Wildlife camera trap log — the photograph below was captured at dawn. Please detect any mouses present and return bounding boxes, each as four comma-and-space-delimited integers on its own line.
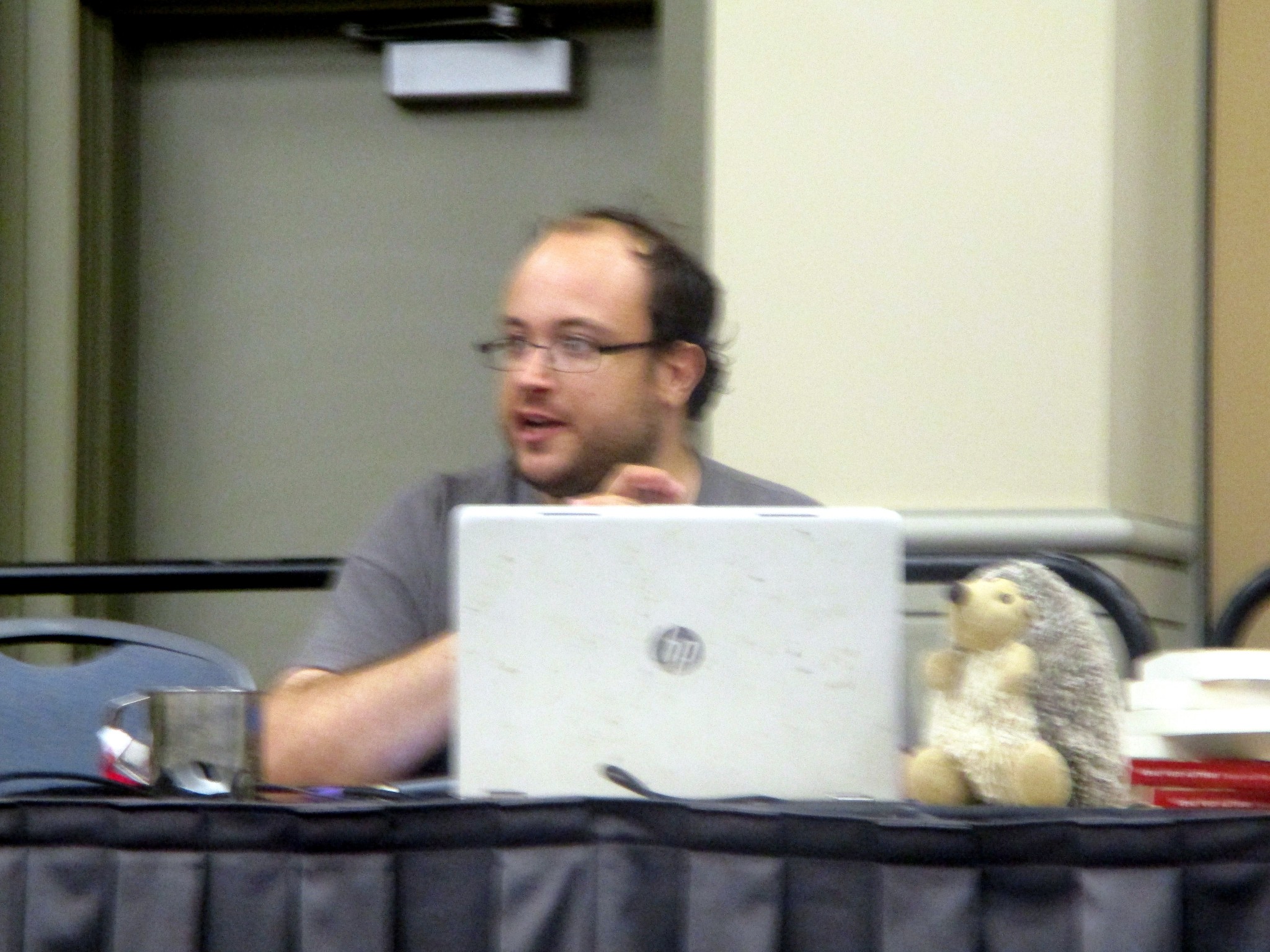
159, 761, 232, 797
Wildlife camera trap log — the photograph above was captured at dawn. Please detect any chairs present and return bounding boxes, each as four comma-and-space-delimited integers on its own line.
0, 615, 258, 794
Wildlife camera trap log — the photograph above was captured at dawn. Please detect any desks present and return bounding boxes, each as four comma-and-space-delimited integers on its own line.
0, 775, 1270, 952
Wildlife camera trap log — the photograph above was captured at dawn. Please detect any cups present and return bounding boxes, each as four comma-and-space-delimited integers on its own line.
105, 687, 256, 794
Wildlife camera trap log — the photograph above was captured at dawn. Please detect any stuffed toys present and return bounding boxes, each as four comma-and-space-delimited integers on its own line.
900, 561, 1134, 814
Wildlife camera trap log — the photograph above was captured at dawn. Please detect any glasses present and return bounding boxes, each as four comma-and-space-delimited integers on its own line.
468, 331, 656, 374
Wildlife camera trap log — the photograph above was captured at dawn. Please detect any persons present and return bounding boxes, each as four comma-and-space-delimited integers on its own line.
255, 207, 917, 804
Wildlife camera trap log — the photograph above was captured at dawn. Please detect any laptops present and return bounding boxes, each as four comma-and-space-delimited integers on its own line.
424, 500, 908, 806
